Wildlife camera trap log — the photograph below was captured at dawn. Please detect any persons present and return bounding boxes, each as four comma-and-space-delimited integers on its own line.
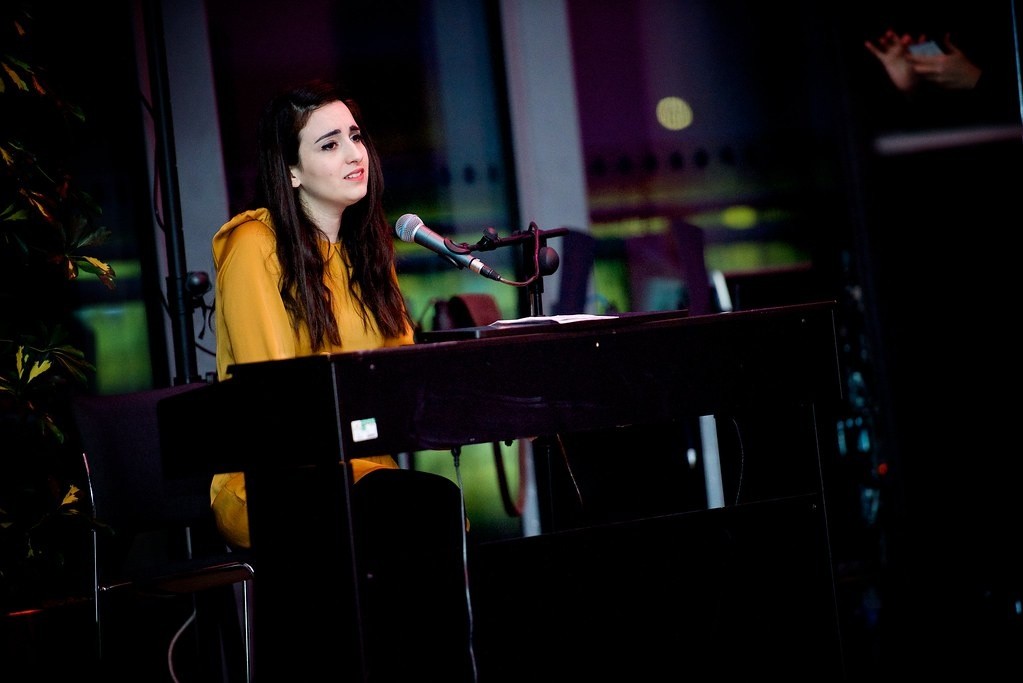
210, 79, 469, 549
857, 1, 1023, 614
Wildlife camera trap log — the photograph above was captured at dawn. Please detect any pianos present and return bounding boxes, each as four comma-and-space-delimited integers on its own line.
145, 294, 867, 464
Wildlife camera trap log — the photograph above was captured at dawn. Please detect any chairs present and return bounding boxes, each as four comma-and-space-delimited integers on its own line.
75, 381, 254, 683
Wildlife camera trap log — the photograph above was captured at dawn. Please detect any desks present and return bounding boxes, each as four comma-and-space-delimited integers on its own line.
166, 296, 843, 683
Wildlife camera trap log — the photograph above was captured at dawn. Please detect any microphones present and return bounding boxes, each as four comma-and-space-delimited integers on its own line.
395, 214, 501, 281
